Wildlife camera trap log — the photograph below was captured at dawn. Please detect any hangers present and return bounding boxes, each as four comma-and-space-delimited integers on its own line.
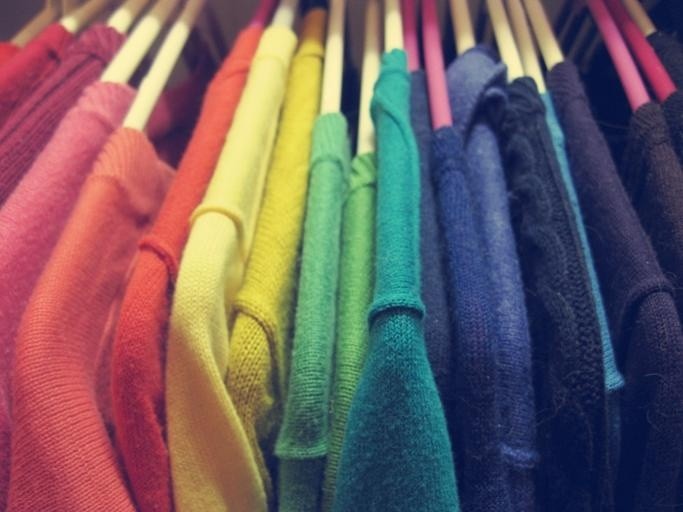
0, 0, 683, 174
